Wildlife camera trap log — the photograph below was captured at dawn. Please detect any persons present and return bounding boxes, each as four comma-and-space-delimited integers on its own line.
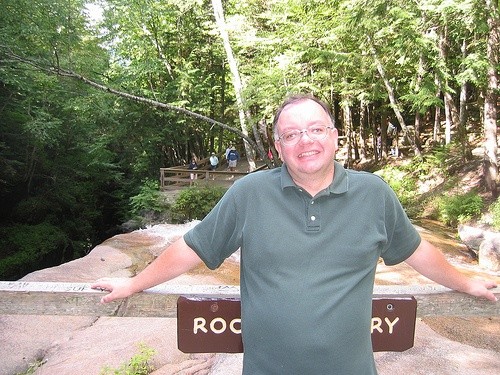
90, 93, 499, 375
225, 144, 233, 166
188, 159, 199, 182
227, 147, 238, 175
377, 121, 396, 156
208, 151, 218, 176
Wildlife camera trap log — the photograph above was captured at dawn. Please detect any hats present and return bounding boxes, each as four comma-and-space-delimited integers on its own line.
212, 152, 215, 155
230, 147, 236, 150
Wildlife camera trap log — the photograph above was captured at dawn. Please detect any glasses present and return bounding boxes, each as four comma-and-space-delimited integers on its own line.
278, 124, 334, 145
